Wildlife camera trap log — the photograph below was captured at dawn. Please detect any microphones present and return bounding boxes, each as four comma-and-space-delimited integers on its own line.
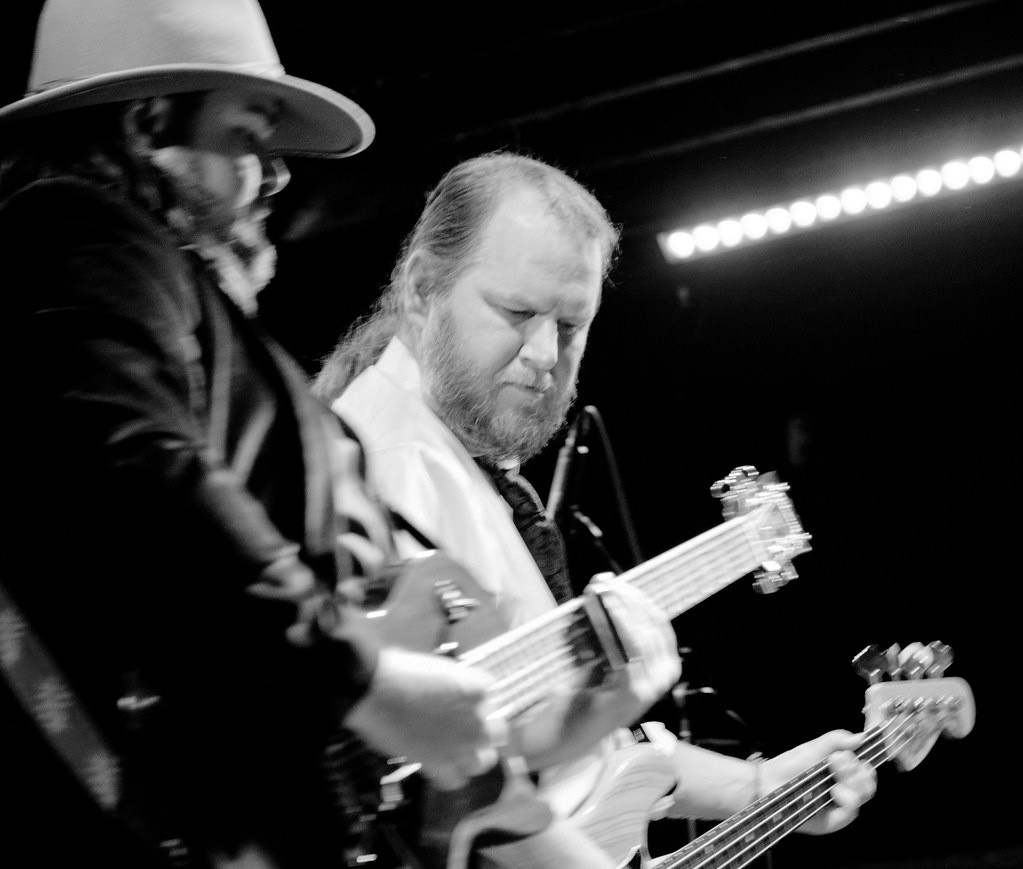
546, 411, 593, 538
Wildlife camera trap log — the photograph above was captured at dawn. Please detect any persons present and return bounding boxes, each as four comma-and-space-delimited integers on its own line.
1, 0, 683, 868
309, 151, 879, 869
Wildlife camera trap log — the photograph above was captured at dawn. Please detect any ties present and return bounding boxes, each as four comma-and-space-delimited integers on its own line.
472, 456, 575, 606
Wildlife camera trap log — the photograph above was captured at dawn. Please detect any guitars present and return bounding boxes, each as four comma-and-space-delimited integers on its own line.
117, 463, 814, 869
567, 637, 976, 869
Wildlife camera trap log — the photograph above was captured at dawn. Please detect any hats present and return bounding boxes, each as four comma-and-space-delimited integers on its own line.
0, 1, 376, 160
